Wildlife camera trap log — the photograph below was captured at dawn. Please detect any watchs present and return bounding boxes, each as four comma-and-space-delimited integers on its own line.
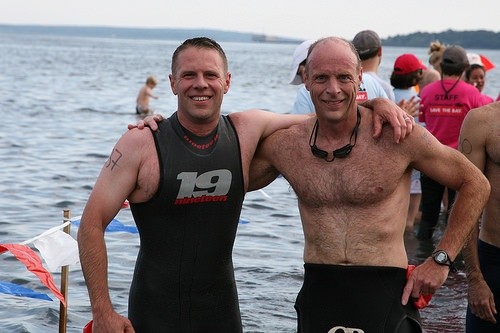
430, 249, 454, 268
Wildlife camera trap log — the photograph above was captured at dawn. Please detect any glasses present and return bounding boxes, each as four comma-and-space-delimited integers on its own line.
310, 143, 355, 159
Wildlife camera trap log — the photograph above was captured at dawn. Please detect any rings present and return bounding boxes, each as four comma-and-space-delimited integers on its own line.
404, 116, 410, 120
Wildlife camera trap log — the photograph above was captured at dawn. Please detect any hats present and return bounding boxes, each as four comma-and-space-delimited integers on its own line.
440, 44, 471, 70
392, 53, 427, 76
466, 53, 485, 67
352, 29, 381, 55
288, 39, 318, 85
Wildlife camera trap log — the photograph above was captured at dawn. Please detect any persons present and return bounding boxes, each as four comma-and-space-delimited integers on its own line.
76, 38, 415, 333
136, 76, 159, 115
127, 37, 493, 333
287, 30, 500, 333
